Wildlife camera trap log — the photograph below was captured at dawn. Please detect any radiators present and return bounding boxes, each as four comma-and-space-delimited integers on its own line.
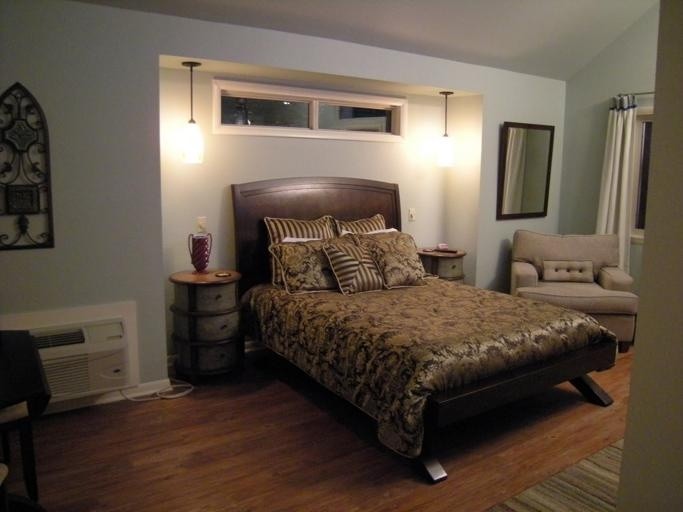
0, 316, 138, 405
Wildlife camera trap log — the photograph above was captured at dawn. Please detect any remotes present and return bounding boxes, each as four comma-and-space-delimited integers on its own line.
434, 248, 457, 253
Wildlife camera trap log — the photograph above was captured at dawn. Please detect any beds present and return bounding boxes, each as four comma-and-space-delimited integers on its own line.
232, 176, 619, 482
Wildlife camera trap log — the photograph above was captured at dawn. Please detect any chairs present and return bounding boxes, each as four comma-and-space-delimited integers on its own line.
510, 228, 639, 353
1, 329, 52, 512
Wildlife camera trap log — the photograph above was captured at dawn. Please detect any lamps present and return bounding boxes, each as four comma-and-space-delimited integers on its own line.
428, 91, 455, 167
165, 60, 203, 165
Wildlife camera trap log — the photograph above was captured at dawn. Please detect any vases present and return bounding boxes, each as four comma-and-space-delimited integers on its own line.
187, 232, 212, 275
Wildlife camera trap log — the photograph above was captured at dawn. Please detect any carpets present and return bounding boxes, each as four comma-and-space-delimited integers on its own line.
483, 438, 625, 512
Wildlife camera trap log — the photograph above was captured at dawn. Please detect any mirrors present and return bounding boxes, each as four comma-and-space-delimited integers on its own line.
496, 122, 554, 220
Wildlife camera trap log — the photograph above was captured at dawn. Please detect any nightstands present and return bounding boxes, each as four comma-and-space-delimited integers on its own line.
169, 271, 240, 391
417, 245, 466, 282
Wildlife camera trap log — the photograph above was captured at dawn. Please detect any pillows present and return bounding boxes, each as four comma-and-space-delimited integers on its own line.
543, 259, 595, 283
347, 230, 418, 247
368, 236, 427, 289
268, 237, 354, 295
322, 243, 387, 296
333, 213, 386, 237
265, 215, 341, 289
